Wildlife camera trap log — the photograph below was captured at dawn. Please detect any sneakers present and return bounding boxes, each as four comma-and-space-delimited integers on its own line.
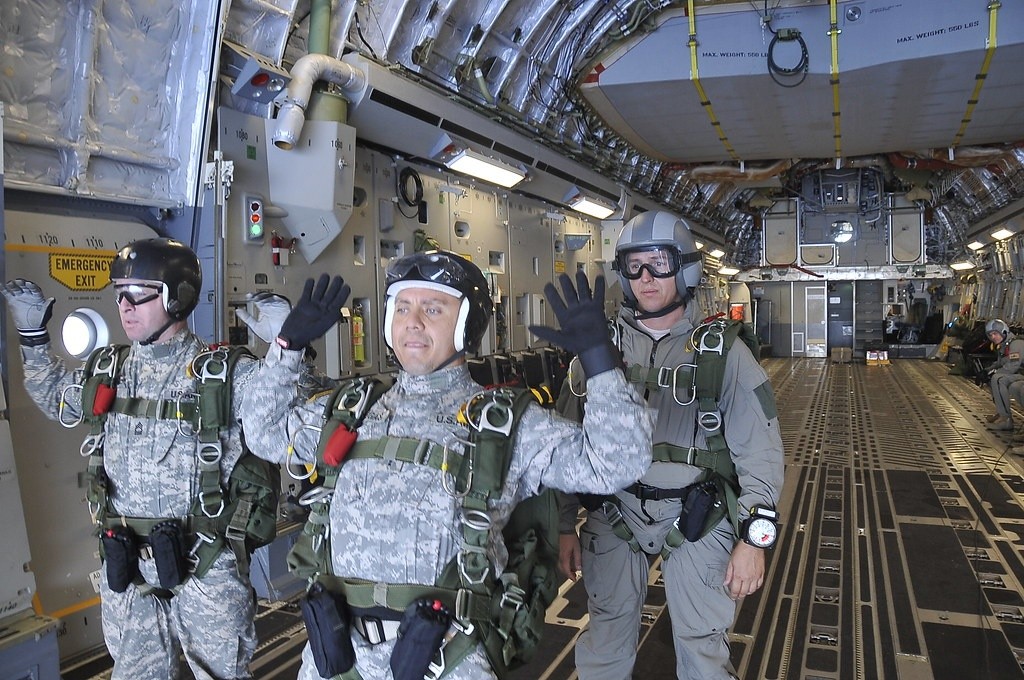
985, 414, 1014, 430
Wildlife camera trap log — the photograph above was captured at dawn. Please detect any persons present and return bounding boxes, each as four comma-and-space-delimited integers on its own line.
977, 319, 1023, 429
240, 252, 654, 679
0, 238, 293, 679
557, 211, 784, 680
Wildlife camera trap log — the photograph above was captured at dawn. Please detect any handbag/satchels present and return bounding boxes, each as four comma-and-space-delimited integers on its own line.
150, 520, 188, 590
299, 585, 356, 680
678, 477, 729, 543
98, 524, 139, 593
389, 598, 452, 680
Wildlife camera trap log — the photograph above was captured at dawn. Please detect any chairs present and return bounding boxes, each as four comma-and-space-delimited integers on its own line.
970, 353, 997, 370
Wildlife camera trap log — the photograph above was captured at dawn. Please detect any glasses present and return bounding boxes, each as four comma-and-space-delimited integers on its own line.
114, 283, 163, 306
385, 254, 495, 314
618, 244, 680, 280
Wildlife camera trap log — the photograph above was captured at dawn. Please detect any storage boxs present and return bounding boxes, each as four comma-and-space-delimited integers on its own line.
830, 347, 852, 362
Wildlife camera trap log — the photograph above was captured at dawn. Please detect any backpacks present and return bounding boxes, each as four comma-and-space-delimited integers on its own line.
78, 341, 281, 551
285, 374, 563, 680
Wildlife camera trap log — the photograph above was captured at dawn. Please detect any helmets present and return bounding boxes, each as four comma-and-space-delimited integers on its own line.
613, 211, 703, 303
985, 319, 1010, 341
109, 238, 203, 323
382, 249, 494, 355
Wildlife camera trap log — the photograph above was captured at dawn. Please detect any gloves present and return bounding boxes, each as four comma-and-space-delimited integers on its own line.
278, 273, 351, 352
528, 271, 624, 380
0, 278, 57, 346
235, 291, 318, 360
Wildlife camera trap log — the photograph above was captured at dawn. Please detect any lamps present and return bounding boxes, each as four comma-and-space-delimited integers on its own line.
569, 196, 615, 219
717, 266, 740, 276
445, 148, 526, 188
949, 260, 977, 270
